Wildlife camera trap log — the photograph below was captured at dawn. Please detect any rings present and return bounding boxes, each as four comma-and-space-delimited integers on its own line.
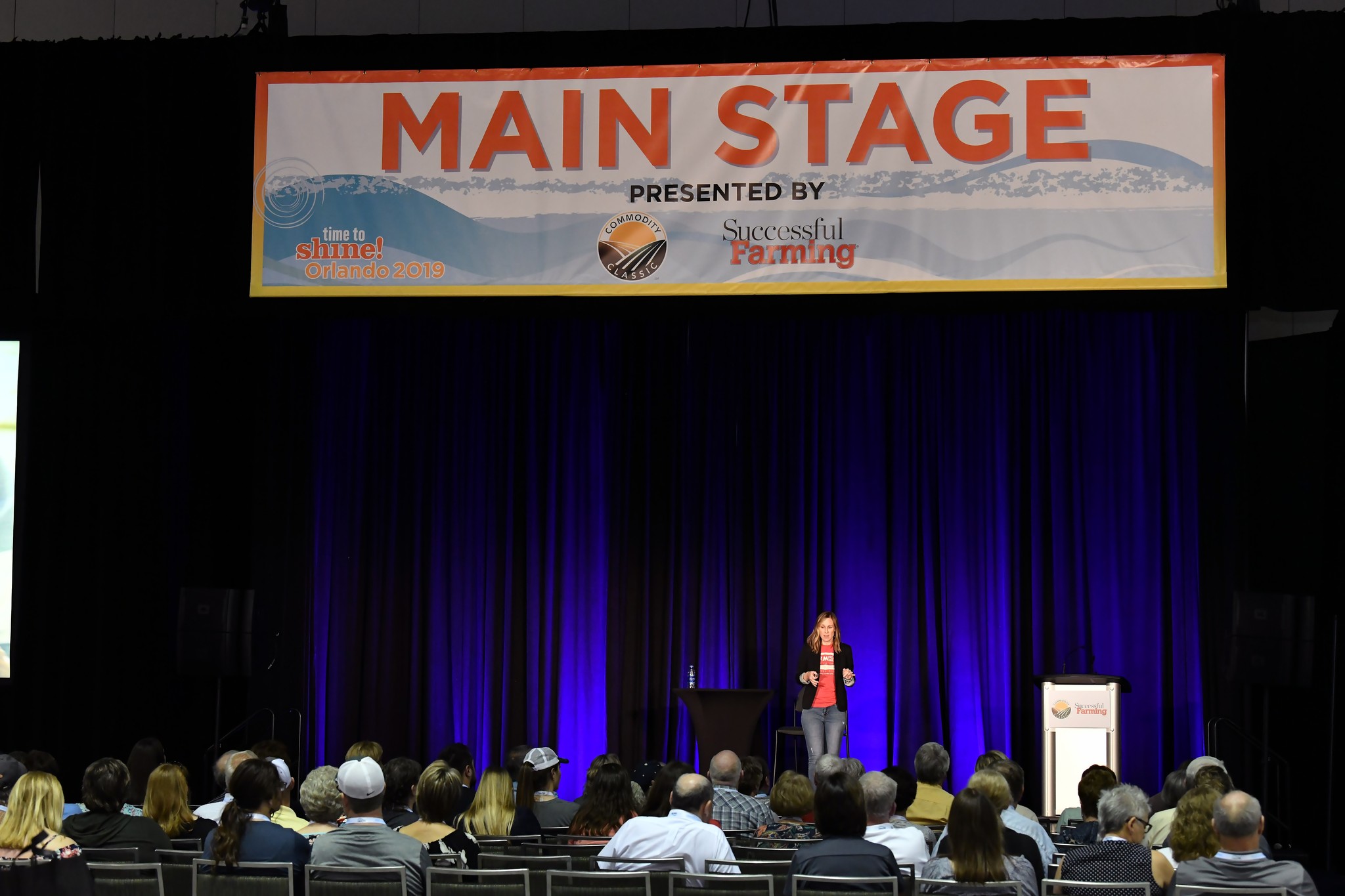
851, 672, 853, 674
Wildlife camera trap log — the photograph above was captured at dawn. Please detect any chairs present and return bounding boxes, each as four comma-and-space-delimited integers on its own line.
0, 808, 1290, 896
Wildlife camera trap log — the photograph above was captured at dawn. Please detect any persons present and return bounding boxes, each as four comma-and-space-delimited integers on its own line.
0, 741, 308, 896
568, 741, 1061, 896
1051, 754, 1319, 896
795, 612, 856, 790
296, 739, 580, 896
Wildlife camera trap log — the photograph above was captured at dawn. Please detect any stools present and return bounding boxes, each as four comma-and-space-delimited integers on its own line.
771, 701, 851, 787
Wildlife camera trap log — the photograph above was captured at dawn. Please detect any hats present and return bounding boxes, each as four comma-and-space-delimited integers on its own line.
264, 756, 291, 790
335, 756, 385, 799
523, 747, 570, 771
1186, 756, 1228, 781
0, 754, 26, 790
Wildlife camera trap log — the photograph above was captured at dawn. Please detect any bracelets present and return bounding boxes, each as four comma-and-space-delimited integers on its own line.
844, 677, 853, 683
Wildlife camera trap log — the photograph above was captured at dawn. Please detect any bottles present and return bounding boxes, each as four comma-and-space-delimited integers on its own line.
688, 665, 695, 689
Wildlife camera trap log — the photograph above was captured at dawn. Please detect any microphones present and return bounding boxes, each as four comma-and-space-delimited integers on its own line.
1063, 646, 1085, 674
268, 659, 275, 669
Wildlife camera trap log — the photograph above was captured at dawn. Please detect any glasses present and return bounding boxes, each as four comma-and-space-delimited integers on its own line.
159, 761, 183, 769
1126, 817, 1153, 833
276, 781, 287, 791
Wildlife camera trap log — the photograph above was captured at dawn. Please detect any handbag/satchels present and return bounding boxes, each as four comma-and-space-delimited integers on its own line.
0, 830, 94, 896
